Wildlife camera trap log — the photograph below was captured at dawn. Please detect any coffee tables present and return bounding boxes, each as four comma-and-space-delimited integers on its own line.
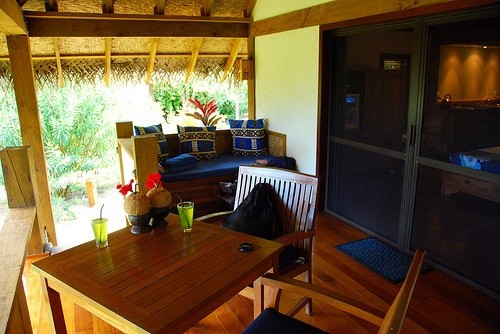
443, 146, 500, 202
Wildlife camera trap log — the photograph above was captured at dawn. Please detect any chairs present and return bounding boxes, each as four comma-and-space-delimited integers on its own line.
195, 164, 320, 317
242, 249, 427, 334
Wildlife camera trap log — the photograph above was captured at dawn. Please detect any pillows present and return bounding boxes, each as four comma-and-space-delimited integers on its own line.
166, 154, 197, 172
176, 125, 218, 161
134, 123, 169, 160
228, 118, 268, 157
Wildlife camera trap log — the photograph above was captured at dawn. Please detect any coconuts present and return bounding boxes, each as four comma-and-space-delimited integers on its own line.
146, 186, 171, 208
124, 191, 151, 217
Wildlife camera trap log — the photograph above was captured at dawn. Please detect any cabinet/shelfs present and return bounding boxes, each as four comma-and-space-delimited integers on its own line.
348, 70, 405, 167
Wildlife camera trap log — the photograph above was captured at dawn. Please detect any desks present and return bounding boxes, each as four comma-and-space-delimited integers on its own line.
32, 213, 283, 334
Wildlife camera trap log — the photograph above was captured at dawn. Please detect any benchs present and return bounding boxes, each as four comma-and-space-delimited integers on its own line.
440, 107, 500, 150
116, 122, 298, 209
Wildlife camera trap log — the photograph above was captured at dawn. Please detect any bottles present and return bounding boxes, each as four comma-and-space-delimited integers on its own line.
436, 97, 442, 108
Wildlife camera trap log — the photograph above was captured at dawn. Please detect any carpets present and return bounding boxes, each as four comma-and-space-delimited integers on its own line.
333, 236, 438, 284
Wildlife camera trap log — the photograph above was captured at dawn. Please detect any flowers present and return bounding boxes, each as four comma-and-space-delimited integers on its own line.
145, 173, 163, 189
117, 179, 135, 194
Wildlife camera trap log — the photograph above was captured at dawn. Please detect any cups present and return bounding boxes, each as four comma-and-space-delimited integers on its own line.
177, 202, 194, 232
90, 217, 109, 248
